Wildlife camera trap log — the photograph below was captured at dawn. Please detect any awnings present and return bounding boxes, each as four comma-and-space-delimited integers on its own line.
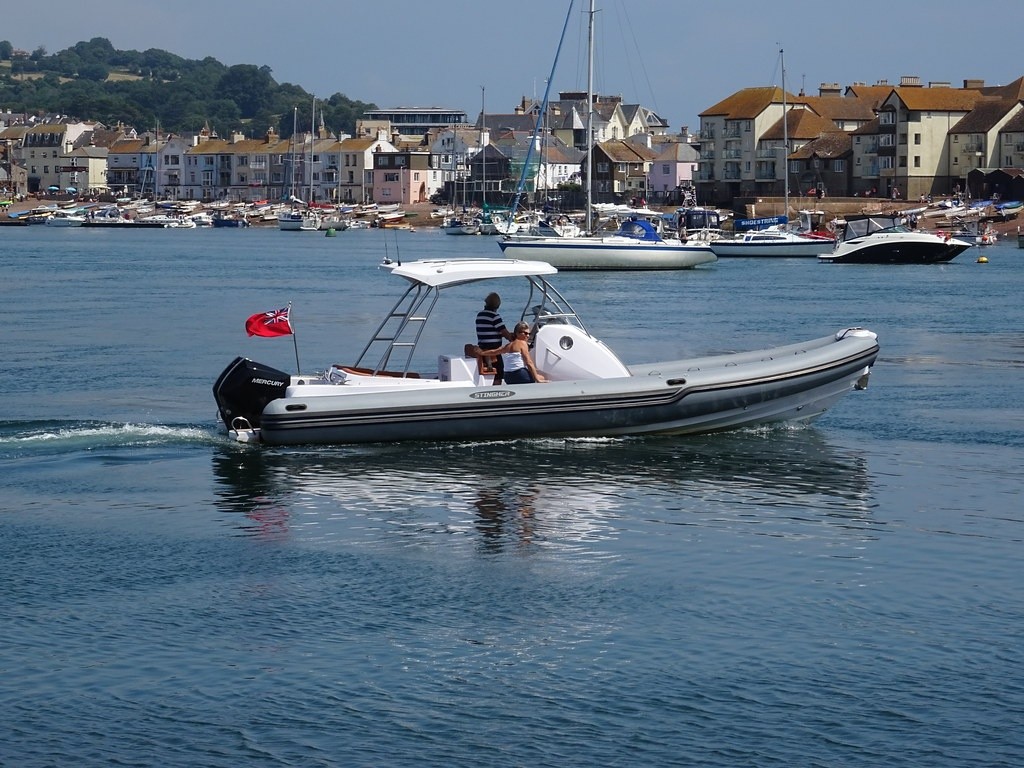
164, 170, 178, 177
106, 167, 138, 171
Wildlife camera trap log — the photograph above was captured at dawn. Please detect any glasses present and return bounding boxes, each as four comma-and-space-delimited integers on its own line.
519, 331, 531, 336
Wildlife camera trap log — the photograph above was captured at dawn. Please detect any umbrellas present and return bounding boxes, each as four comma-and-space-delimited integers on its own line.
99, 185, 111, 194
49, 185, 59, 194
123, 185, 129, 197
66, 187, 77, 195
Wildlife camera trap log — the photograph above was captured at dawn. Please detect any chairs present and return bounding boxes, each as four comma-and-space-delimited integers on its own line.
466, 344, 498, 375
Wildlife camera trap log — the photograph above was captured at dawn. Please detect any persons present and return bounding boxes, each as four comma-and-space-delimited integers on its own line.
892, 190, 897, 201
863, 186, 866, 198
473, 321, 550, 384
954, 183, 962, 206
2, 186, 15, 214
475, 292, 514, 385
91, 209, 94, 219
93, 187, 99, 196
893, 209, 917, 229
927, 193, 931, 203
872, 187, 877, 198
115, 189, 123, 198
920, 192, 925, 204
815, 187, 822, 202
640, 197, 646, 208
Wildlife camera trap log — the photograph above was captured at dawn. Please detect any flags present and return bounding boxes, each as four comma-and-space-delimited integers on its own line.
246, 312, 293, 338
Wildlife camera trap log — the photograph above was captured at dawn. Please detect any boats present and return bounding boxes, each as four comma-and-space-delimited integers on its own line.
430, 0, 1024, 268
0, 94, 417, 230
212, 233, 881, 447
